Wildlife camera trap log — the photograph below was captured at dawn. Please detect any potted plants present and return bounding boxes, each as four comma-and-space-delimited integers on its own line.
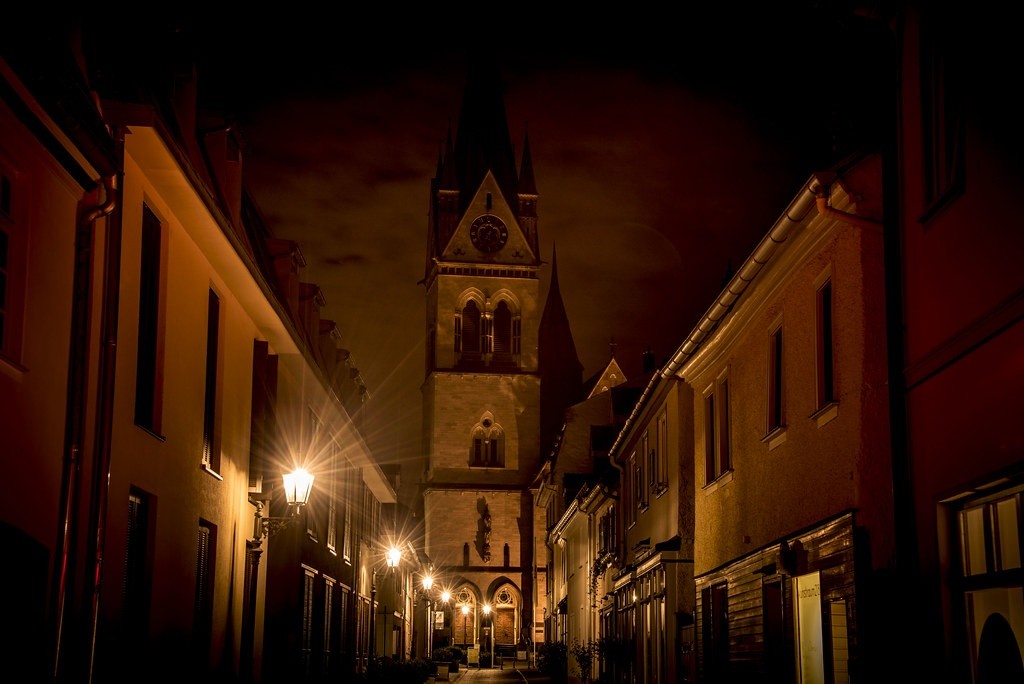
432, 648, 453, 680
444, 646, 465, 673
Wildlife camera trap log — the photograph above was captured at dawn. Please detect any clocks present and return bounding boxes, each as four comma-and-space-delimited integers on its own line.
471, 216, 508, 253
